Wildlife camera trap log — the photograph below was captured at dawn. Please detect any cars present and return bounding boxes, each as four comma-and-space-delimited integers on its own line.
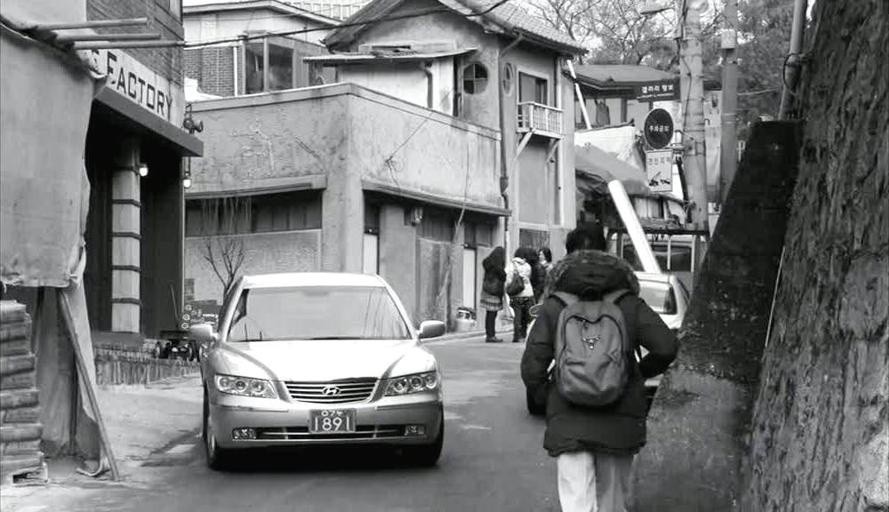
187, 268, 446, 468
521, 267, 691, 418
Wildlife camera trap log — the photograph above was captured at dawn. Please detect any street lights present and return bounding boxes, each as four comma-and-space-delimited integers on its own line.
634, 3, 711, 256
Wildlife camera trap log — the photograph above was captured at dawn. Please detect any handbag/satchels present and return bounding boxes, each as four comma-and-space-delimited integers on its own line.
507, 273, 524, 296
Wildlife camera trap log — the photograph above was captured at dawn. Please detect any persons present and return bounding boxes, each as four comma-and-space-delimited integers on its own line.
479, 247, 553, 341
521, 227, 678, 512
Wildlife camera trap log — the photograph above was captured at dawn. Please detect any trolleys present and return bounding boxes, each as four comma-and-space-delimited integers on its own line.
153, 282, 197, 362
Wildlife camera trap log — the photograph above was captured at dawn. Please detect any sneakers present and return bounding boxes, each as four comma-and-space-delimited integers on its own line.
486, 336, 501, 342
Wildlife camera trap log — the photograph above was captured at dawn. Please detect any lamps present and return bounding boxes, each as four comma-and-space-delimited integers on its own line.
183, 103, 205, 135
128, 137, 149, 179
179, 150, 195, 193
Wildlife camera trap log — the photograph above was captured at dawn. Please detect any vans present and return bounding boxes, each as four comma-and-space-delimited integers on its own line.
614, 229, 692, 297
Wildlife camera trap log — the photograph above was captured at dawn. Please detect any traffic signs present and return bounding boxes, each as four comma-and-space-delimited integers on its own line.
633, 78, 680, 105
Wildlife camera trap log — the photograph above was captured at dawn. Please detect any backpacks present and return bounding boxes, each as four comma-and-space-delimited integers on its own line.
549, 289, 631, 406
